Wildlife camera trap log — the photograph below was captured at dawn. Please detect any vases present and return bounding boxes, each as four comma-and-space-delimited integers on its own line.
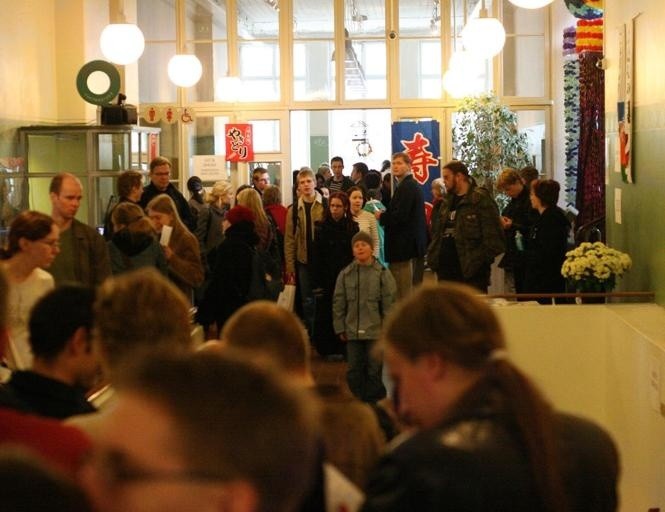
561, 241, 633, 286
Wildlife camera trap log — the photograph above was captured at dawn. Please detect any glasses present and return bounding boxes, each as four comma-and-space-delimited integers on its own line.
167, 47, 202, 88
443, 0, 506, 99
98, 14, 145, 66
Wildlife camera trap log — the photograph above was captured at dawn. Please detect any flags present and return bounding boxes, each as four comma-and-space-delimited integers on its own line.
578, 278, 609, 303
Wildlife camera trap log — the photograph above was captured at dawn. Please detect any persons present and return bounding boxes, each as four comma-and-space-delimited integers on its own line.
1, 281, 101, 421
80, 349, 314, 510
103, 153, 572, 396
2, 443, 94, 512
2, 209, 61, 369
223, 300, 386, 492
39, 170, 114, 289
358, 281, 621, 510
62, 272, 194, 435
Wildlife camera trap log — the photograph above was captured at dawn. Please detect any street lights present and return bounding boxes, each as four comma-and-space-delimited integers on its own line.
278, 283, 296, 313
425, 233, 459, 275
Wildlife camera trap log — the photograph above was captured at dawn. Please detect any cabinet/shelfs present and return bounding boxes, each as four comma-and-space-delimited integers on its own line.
515, 230, 523, 252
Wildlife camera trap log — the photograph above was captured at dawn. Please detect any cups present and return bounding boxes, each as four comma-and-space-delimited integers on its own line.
151, 171, 170, 176
